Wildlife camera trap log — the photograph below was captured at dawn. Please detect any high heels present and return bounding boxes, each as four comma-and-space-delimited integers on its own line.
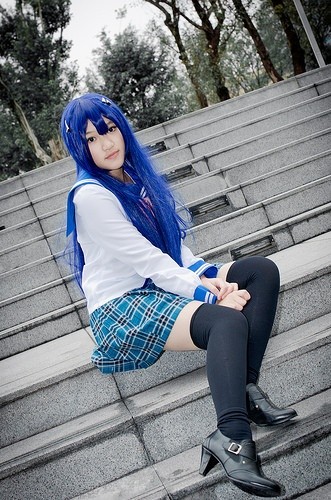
245, 382, 297, 428
198, 428, 281, 498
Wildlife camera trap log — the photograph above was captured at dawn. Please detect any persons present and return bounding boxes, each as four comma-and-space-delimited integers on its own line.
59, 91, 301, 497
